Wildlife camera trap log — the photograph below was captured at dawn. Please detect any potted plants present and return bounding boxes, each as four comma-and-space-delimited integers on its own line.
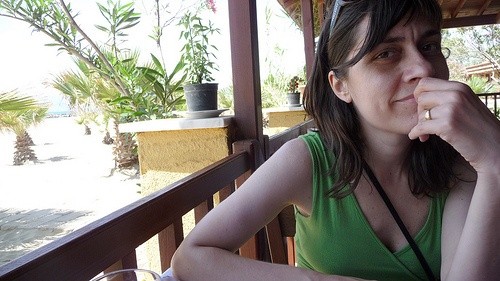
284, 76, 304, 104
177, 11, 222, 111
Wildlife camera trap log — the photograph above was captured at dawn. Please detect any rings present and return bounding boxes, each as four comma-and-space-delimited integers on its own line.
425, 110, 432, 120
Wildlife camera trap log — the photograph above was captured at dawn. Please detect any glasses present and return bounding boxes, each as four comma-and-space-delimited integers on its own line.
329, 0, 356, 39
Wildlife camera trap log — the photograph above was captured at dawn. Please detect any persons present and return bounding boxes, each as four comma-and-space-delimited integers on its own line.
171, 0, 500, 281
